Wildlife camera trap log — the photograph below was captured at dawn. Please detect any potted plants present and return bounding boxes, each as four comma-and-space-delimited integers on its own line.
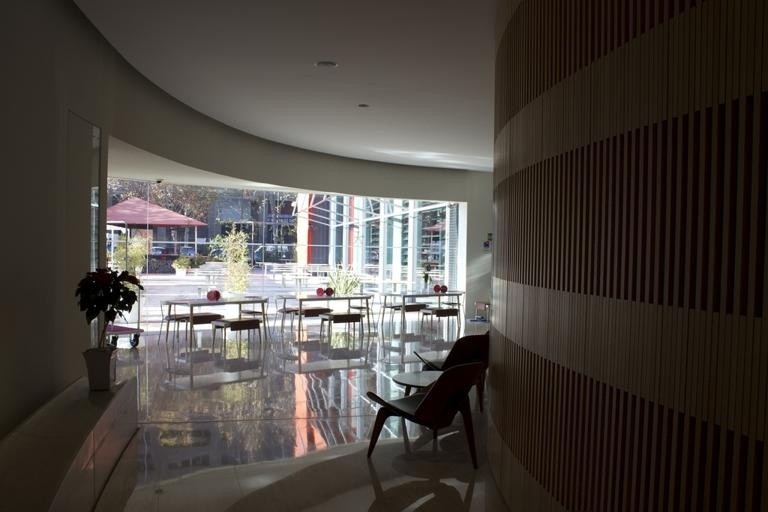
171, 255, 191, 276
74, 267, 144, 391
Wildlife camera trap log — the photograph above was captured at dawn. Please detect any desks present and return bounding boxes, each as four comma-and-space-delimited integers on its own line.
474, 299, 490, 322
196, 259, 440, 293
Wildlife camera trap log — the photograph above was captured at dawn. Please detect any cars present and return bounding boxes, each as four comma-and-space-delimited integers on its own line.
209, 248, 224, 256
181, 247, 194, 255
151, 246, 164, 255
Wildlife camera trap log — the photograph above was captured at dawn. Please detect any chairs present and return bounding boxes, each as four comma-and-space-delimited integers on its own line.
380, 331, 459, 358
239, 296, 269, 330
417, 329, 490, 410
366, 362, 482, 470
392, 335, 484, 448
377, 291, 463, 331
273, 334, 376, 374
157, 299, 188, 345
273, 293, 374, 333
161, 344, 269, 391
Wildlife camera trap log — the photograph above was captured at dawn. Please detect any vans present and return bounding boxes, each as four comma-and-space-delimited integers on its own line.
254, 243, 293, 263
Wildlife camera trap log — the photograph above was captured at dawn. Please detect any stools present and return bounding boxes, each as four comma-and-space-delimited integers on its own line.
211, 319, 262, 347
165, 312, 224, 340
